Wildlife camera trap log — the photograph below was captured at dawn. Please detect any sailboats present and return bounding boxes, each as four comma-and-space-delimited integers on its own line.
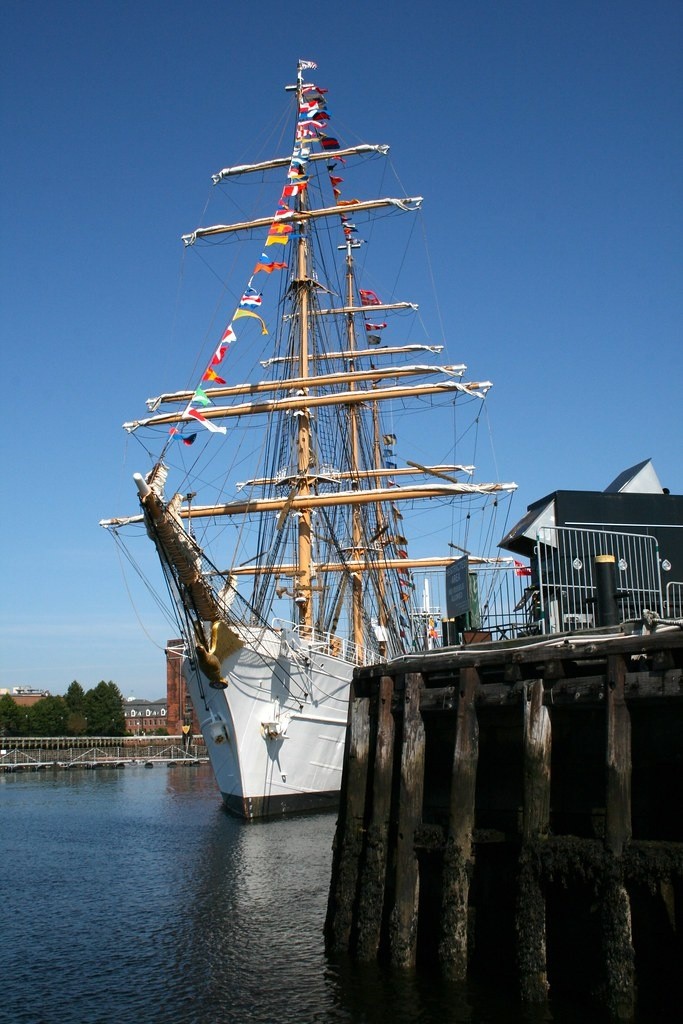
96, 58, 541, 821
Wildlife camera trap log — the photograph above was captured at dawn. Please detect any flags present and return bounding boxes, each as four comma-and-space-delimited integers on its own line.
300, 60, 318, 71
372, 368, 382, 384
167, 253, 289, 447
366, 322, 387, 331
430, 629, 438, 639
385, 461, 397, 469
382, 449, 392, 458
430, 618, 437, 628
361, 289, 382, 305
514, 561, 532, 576
383, 434, 397, 446
392, 505, 413, 637
388, 479, 400, 488
264, 93, 370, 246
368, 334, 381, 345
